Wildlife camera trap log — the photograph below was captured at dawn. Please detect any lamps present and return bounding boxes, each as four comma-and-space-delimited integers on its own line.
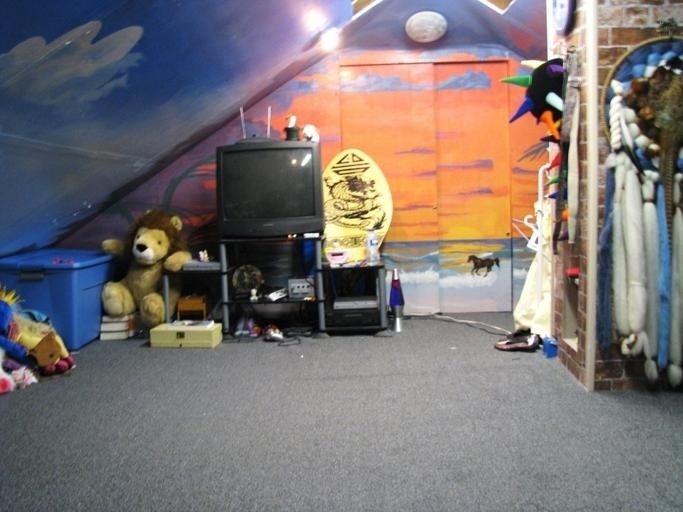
404, 11, 448, 42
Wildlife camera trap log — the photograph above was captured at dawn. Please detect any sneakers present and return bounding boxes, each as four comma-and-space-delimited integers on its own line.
494, 335, 539, 352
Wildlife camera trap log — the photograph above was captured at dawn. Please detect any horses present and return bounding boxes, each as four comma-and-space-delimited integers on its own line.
468, 255, 500, 277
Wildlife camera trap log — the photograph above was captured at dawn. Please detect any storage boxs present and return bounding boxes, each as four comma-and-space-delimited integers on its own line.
0, 247, 118, 351
147, 321, 223, 349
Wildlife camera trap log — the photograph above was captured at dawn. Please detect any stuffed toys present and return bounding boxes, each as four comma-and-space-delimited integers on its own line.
102, 209, 193, 329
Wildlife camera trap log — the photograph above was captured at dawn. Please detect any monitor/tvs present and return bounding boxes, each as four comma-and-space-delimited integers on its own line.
216, 142, 325, 238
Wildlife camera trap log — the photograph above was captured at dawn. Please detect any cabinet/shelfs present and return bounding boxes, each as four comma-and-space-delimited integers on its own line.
162, 236, 403, 337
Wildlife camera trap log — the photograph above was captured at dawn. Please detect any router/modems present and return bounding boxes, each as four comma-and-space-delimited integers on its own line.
236, 106, 276, 144
